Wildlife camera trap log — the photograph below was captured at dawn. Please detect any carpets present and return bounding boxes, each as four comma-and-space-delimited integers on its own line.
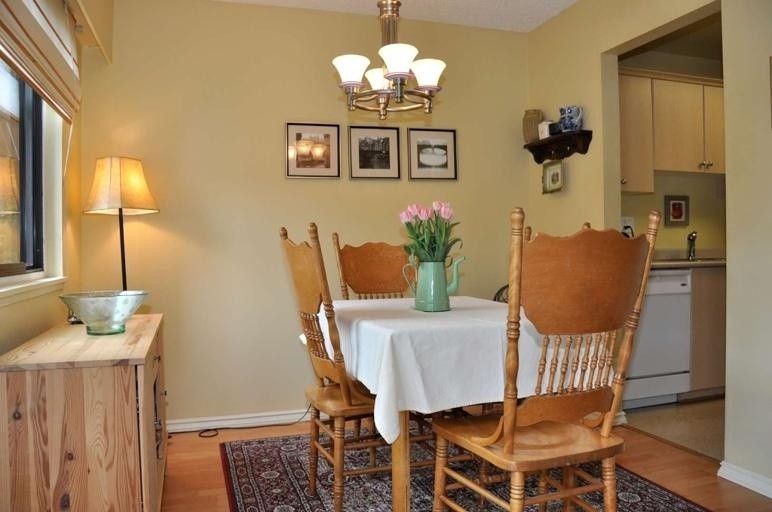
218, 418, 714, 512
619, 396, 724, 463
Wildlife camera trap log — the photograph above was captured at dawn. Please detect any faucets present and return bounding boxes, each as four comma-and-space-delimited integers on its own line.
687, 230, 697, 259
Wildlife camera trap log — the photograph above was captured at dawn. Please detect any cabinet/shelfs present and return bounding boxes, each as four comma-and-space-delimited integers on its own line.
1, 312, 170, 512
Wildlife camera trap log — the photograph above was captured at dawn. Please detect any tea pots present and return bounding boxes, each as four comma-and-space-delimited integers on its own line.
401, 257, 464, 312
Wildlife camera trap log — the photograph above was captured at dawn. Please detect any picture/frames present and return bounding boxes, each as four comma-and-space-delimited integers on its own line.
664, 195, 689, 226
541, 158, 564, 195
286, 121, 341, 178
347, 124, 400, 179
407, 127, 457, 179
651, 78, 725, 175
617, 73, 654, 194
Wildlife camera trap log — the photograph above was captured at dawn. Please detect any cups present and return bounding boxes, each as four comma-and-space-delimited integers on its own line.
559, 106, 583, 133
522, 109, 544, 145
296, 139, 329, 161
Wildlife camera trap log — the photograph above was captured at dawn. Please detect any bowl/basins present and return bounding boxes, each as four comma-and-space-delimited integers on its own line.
58, 291, 149, 336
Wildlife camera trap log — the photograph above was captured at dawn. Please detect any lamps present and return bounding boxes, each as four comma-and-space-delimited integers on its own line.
288, 139, 326, 163
332, 0, 446, 119
81, 155, 159, 290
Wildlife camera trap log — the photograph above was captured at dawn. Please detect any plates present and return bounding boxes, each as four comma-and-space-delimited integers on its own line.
419, 147, 447, 167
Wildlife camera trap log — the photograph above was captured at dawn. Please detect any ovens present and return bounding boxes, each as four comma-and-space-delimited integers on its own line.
612, 267, 692, 400
